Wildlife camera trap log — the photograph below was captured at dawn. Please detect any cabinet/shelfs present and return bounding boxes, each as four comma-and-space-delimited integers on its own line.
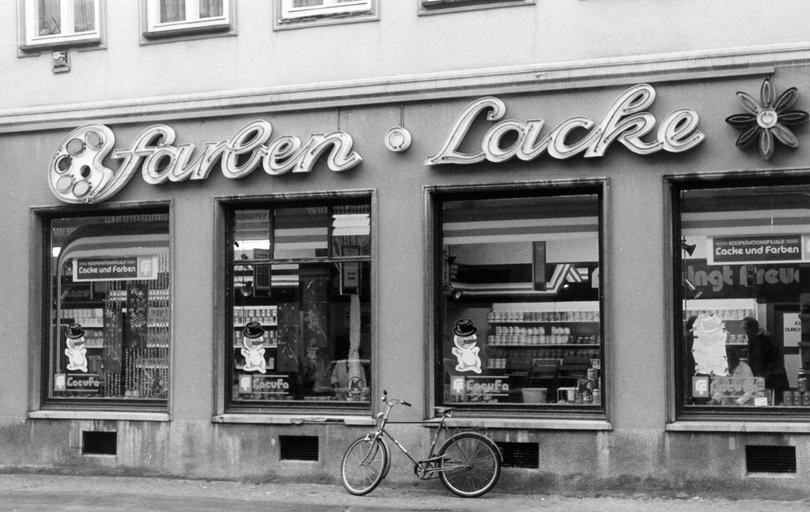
485, 297, 759, 389
60, 300, 105, 374
232, 305, 277, 370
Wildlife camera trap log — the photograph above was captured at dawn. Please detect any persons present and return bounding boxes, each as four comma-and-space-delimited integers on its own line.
741, 315, 791, 403
331, 346, 367, 403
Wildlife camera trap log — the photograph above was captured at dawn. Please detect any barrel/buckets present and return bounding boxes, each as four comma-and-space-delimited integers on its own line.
522, 387, 548, 404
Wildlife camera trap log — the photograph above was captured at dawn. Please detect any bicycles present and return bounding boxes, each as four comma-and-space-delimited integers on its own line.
340, 389, 503, 498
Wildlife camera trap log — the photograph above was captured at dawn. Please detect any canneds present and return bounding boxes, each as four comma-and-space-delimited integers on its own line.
233, 330, 278, 346
487, 349, 601, 369
487, 311, 600, 323
575, 368, 601, 405
268, 357, 275, 370
233, 309, 278, 327
62, 308, 104, 326
487, 326, 601, 345
783, 370, 809, 406
83, 329, 103, 349
